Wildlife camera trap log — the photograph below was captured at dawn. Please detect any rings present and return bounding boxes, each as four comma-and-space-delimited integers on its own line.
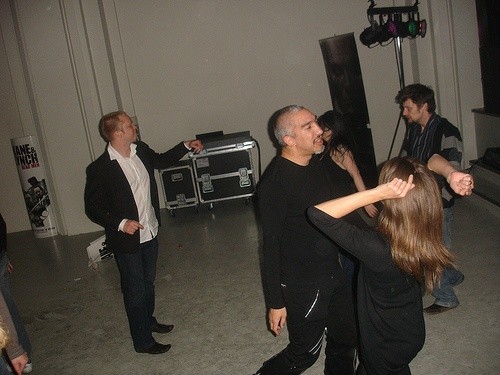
469, 181, 472, 185
470, 186, 473, 190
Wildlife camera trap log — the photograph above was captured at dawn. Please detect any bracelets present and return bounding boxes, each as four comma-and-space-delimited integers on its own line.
447, 169, 457, 183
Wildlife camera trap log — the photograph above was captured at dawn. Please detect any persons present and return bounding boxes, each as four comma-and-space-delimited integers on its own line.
23, 177, 49, 228
84, 111, 203, 354
251, 105, 359, 375
395, 83, 465, 315
311, 110, 378, 218
0, 212, 32, 375
306, 153, 474, 375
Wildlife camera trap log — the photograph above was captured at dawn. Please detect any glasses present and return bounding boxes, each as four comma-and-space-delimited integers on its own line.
323, 128, 331, 135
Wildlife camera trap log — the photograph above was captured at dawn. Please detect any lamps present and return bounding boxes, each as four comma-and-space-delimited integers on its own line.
359, 5, 427, 49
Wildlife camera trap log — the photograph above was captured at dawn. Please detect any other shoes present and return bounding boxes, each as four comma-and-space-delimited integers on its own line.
423, 303, 451, 315
23, 362, 32, 374
152, 323, 174, 333
136, 342, 171, 354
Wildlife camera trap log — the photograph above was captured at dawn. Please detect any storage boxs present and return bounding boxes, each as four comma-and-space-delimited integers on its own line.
158, 148, 256, 216
